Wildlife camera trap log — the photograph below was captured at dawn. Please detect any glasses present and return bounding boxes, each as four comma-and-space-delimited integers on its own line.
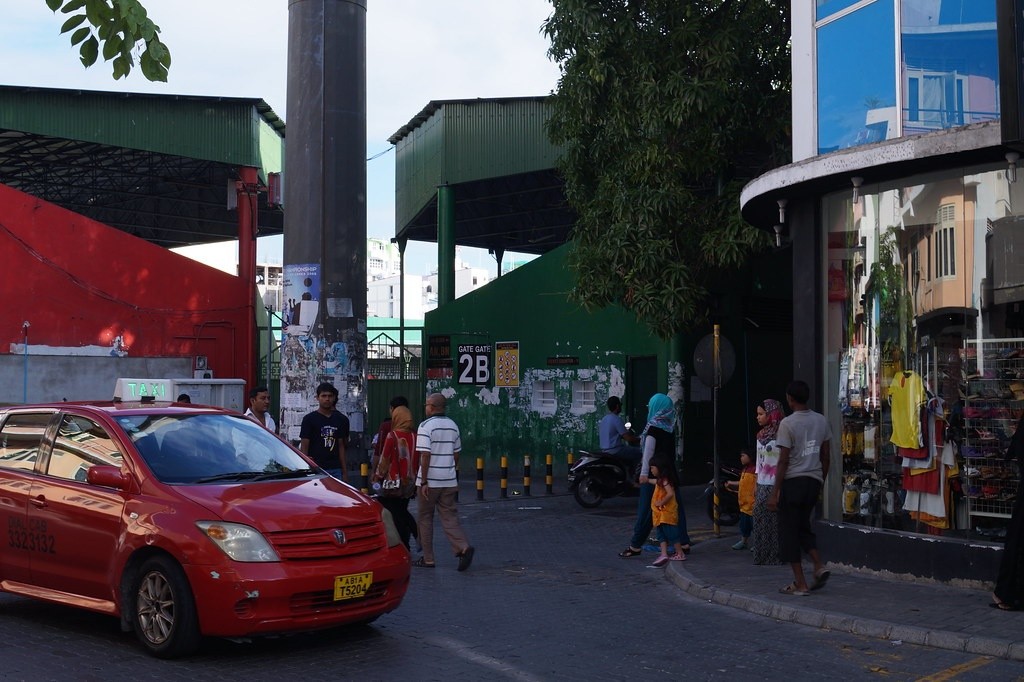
424, 403, 436, 408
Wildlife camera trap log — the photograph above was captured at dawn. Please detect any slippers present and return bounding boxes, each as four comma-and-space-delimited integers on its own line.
989, 602, 1016, 611
810, 570, 830, 590
414, 556, 436, 567
456, 547, 475, 571
779, 581, 810, 596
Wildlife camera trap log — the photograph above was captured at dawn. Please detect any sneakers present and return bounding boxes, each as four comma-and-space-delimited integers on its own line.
669, 554, 687, 561
653, 554, 668, 566
732, 542, 748, 550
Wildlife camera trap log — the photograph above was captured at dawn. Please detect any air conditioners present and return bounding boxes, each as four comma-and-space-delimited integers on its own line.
194, 370, 213, 379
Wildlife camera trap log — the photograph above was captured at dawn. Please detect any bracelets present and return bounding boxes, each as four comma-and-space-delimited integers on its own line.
420, 481, 427, 487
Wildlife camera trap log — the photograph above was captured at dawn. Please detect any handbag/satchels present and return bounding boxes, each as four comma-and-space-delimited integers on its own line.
378, 478, 415, 499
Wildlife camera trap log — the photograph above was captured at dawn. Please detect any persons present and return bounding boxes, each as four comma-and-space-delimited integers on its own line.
370, 406, 422, 553
619, 394, 690, 556
752, 398, 786, 564
411, 393, 474, 572
177, 395, 191, 404
732, 450, 757, 553
371, 397, 408, 474
769, 381, 834, 595
638, 456, 687, 565
989, 411, 1024, 612
298, 382, 345, 484
600, 397, 641, 489
331, 388, 349, 466
232, 386, 276, 472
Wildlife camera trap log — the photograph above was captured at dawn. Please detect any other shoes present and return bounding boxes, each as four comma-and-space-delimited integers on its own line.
958, 348, 1024, 502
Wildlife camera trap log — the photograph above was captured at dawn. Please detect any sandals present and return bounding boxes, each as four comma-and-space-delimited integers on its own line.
681, 548, 690, 555
619, 547, 641, 557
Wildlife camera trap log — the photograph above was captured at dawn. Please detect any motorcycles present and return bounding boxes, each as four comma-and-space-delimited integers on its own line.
704, 461, 741, 525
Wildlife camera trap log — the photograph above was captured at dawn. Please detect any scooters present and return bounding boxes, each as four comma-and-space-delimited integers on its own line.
567, 423, 643, 507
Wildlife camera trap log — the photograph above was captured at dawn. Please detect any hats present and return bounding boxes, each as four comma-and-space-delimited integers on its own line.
649, 452, 674, 470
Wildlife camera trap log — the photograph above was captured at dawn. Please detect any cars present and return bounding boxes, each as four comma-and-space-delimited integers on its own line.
0, 393, 413, 660
